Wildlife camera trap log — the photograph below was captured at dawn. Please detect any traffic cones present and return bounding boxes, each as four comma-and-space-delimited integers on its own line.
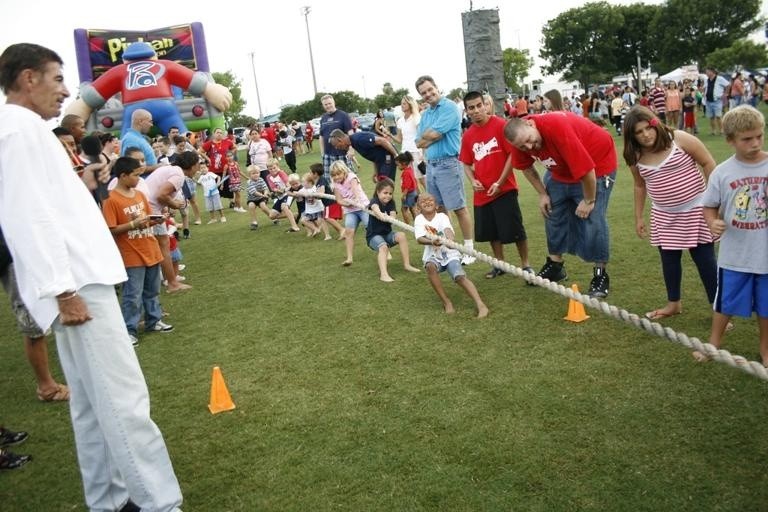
205, 366, 236, 415
560, 284, 590, 323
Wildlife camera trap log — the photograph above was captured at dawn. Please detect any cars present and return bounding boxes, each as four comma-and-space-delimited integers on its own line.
229, 112, 374, 144
543, 64, 767, 110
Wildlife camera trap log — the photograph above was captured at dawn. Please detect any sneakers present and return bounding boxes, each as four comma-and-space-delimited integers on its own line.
527, 257, 568, 287
129, 261, 192, 348
1, 452, 31, 470
590, 266, 610, 298
251, 217, 300, 233
460, 252, 477, 266
230, 201, 247, 213
0, 428, 28, 449
174, 215, 226, 239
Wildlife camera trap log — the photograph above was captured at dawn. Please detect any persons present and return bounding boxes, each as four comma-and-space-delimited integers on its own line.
503, 110, 618, 299
53, 108, 201, 345
1, 241, 71, 402
413, 194, 490, 318
1, 44, 183, 511
1, 424, 33, 471
457, 91, 535, 286
623, 104, 732, 329
501, 87, 561, 117
563, 67, 765, 137
692, 104, 768, 359
195, 94, 428, 282
415, 76, 476, 257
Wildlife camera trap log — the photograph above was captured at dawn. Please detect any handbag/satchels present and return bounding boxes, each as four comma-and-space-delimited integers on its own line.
205, 142, 212, 167
246, 141, 252, 166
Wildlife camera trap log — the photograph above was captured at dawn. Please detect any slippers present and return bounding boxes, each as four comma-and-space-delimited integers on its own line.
645, 310, 682, 320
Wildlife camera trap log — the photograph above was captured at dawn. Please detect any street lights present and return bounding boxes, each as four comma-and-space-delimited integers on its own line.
248, 48, 264, 122
302, 4, 317, 97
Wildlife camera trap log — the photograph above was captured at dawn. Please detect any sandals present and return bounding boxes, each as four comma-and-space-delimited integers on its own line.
37, 384, 69, 402
486, 267, 505, 279
522, 266, 535, 285
